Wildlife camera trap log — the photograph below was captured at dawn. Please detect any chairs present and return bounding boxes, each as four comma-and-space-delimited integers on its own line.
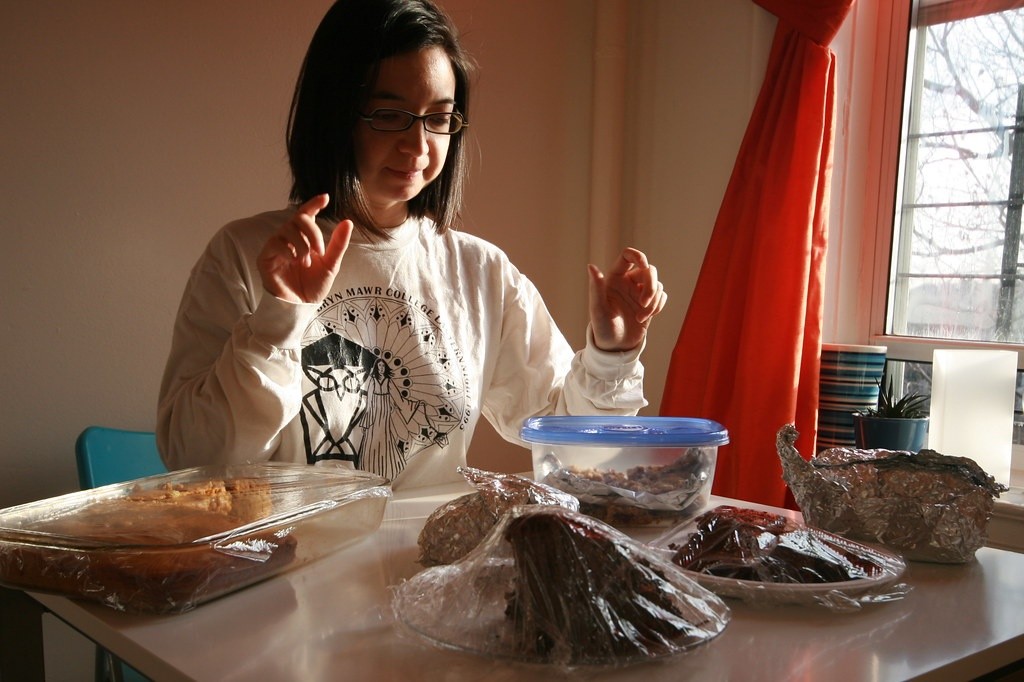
73, 425, 169, 682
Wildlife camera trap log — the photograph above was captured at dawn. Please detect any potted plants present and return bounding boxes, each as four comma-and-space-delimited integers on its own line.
854, 374, 930, 453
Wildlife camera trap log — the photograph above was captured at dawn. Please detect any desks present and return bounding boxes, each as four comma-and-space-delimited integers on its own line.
23, 469, 1024, 682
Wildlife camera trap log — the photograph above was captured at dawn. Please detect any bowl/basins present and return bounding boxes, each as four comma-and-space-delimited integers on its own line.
518, 415, 730, 529
0, 463, 393, 616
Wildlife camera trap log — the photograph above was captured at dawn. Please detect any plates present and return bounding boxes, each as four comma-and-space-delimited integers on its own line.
679, 549, 908, 605
389, 570, 732, 672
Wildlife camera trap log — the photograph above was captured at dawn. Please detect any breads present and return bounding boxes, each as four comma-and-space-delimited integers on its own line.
503, 512, 693, 655
0, 478, 298, 611
543, 447, 711, 524
668, 505, 885, 583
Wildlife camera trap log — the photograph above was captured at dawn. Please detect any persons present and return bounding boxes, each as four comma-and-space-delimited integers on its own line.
153, 0, 667, 491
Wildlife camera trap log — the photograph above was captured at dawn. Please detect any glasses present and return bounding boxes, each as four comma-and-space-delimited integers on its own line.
359, 107, 468, 134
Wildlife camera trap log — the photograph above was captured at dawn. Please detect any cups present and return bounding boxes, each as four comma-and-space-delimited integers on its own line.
815, 342, 887, 457
